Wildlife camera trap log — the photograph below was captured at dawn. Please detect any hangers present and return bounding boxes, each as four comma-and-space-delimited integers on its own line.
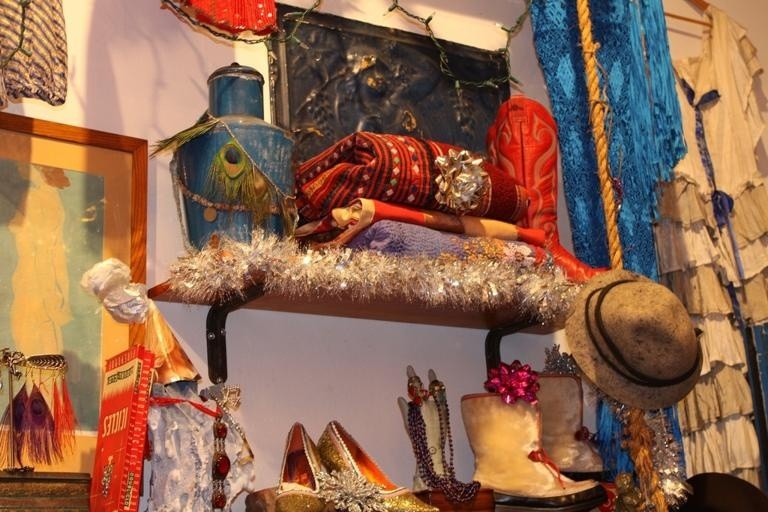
664, 0, 714, 28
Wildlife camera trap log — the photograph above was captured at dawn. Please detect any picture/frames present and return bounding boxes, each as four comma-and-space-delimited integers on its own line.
0, 108, 150, 500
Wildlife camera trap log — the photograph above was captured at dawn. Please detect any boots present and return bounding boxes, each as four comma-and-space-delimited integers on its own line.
459, 390, 610, 512
530, 373, 611, 482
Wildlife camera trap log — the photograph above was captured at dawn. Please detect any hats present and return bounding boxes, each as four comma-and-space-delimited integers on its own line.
564, 266, 704, 412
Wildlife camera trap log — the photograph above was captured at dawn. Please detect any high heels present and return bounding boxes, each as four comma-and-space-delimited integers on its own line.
273, 421, 327, 512
316, 419, 440, 512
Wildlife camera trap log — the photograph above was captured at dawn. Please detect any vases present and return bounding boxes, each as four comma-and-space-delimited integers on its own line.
170, 65, 297, 256
